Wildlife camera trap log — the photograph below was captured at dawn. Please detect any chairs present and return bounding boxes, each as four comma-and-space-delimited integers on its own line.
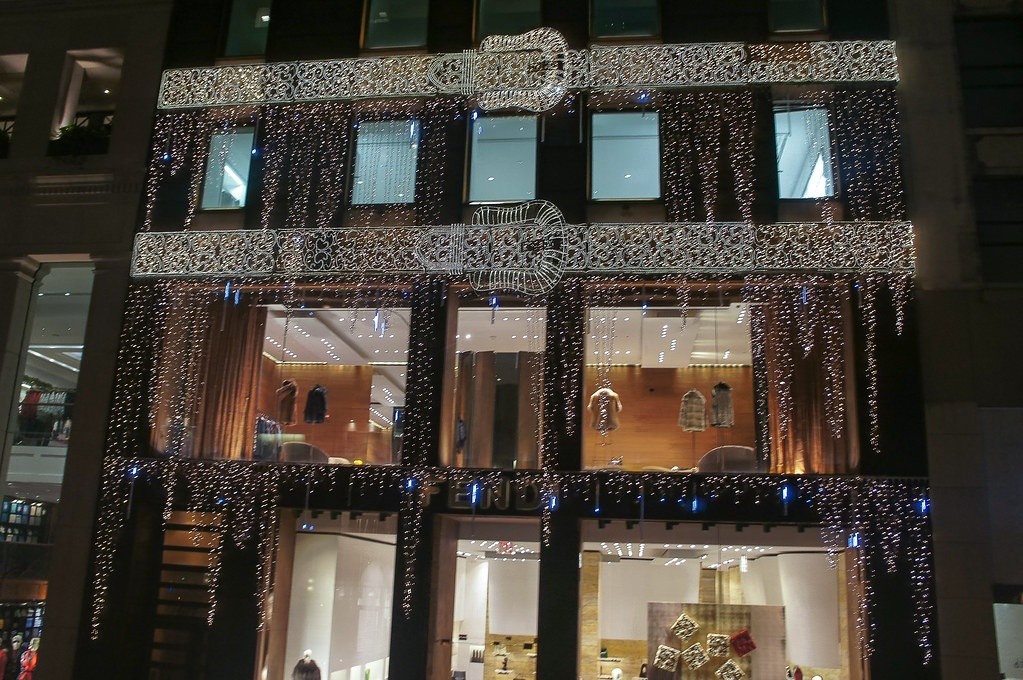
696, 445, 757, 473
283, 442, 329, 463
328, 457, 350, 465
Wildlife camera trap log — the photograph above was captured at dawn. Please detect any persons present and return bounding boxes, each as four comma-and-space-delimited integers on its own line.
293, 650, 321, 680
0, 635, 40, 680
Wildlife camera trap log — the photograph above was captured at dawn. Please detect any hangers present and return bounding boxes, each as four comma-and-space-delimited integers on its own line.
32, 385, 76, 393
258, 408, 277, 423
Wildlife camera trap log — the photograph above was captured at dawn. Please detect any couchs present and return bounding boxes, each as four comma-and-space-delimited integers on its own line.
253, 434, 305, 462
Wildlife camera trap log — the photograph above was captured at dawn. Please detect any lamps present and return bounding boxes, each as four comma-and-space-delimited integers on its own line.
293, 510, 392, 522
